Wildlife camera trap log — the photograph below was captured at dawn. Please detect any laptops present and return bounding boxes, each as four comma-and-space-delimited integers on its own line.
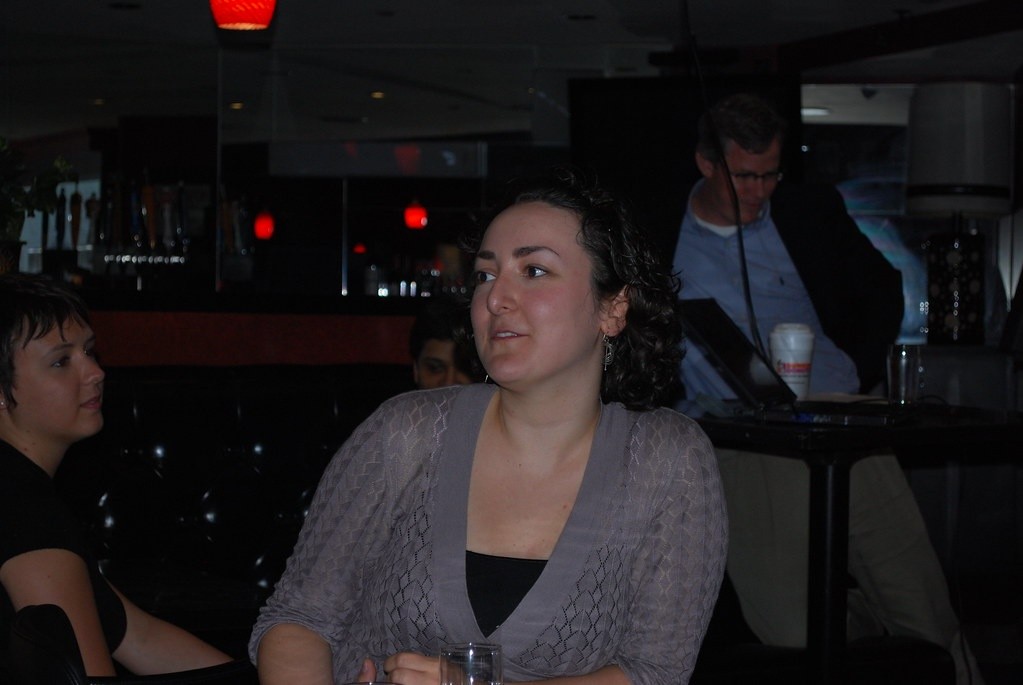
675, 298, 922, 426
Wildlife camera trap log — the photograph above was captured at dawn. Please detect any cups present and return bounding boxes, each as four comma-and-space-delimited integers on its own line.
886, 342, 920, 410
768, 323, 814, 401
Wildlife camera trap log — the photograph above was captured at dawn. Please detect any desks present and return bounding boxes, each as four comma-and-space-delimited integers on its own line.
691, 401, 1023, 685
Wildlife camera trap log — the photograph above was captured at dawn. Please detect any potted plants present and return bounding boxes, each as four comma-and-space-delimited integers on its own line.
0, 137, 72, 275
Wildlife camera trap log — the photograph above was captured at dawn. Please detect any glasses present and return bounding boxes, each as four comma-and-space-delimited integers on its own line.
723, 155, 783, 183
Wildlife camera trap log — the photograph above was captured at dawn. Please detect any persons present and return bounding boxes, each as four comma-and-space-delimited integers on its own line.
0, 273, 234, 685
631, 91, 981, 685
408, 305, 476, 388
246, 170, 729, 685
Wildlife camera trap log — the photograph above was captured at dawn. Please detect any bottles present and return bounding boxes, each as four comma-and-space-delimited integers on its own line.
41, 161, 209, 260
360, 264, 472, 311
